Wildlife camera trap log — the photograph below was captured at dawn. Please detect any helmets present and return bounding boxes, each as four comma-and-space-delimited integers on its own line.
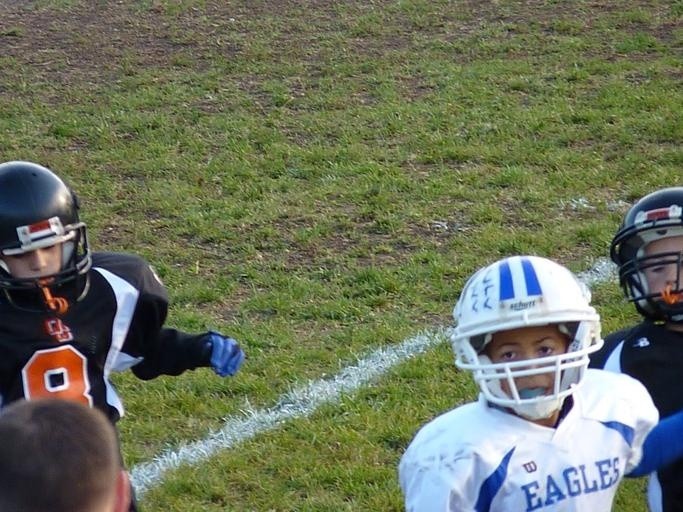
449, 256, 605, 421
0, 160, 92, 314
610, 187, 683, 324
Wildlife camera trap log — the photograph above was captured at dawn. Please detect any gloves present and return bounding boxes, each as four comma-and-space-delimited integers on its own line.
196, 332, 242, 375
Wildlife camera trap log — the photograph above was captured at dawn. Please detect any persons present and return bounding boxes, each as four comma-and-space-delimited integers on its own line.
399, 254, 682, 512
0, 400, 133, 512
584, 187, 682, 511
0, 160, 243, 511
